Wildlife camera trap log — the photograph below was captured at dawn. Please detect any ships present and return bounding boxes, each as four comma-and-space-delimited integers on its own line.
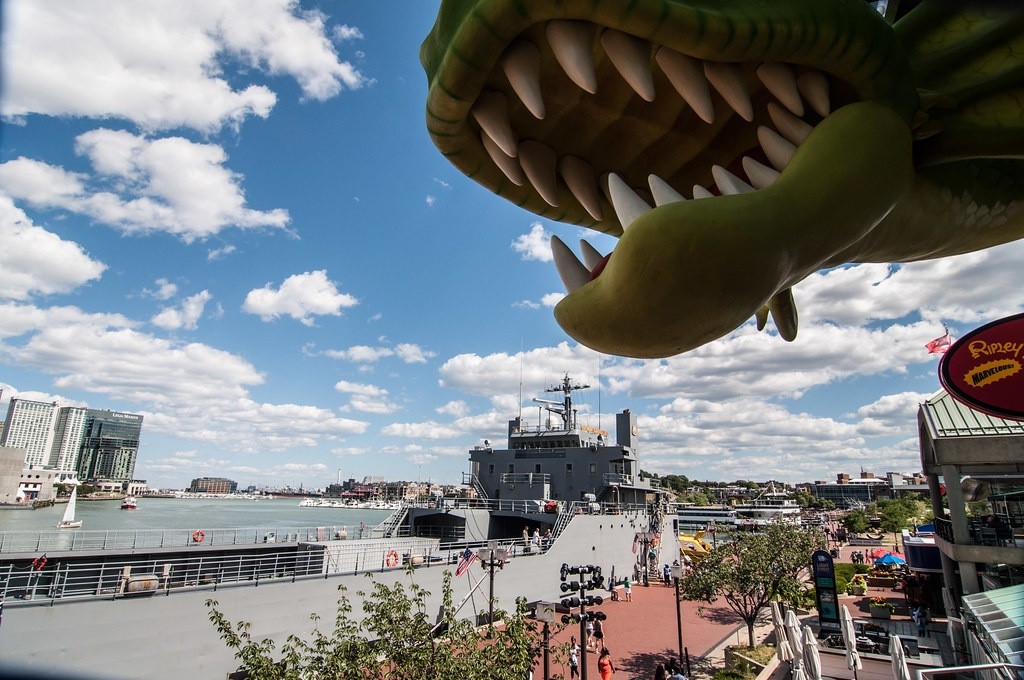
1, 372, 678, 680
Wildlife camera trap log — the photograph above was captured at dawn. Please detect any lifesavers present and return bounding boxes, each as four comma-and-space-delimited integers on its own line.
192, 531, 205, 543
387, 550, 398, 567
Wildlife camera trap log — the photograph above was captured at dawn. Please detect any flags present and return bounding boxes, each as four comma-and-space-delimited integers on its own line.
454, 547, 477, 578
925, 333, 952, 354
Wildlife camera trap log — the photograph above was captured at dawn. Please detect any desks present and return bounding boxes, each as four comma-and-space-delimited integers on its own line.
856, 638, 874, 653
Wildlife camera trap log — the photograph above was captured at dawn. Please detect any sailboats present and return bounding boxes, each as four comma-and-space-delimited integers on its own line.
56, 484, 84, 529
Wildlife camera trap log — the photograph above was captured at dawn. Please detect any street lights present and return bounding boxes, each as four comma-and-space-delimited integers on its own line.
671, 566, 684, 675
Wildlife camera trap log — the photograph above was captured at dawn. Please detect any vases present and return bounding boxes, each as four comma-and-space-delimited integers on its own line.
853, 582, 867, 596
871, 607, 890, 619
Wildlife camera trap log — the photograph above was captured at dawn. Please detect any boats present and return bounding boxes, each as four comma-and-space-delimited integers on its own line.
671, 503, 736, 531
143, 488, 175, 498
299, 497, 401, 509
678, 532, 713, 557
120, 496, 138, 509
733, 482, 804, 536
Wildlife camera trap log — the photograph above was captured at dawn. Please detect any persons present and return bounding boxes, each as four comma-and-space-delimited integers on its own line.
902, 576, 929, 636
851, 548, 874, 564
894, 543, 901, 554
522, 499, 680, 680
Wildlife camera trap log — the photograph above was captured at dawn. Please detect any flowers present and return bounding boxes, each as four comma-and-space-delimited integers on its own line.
847, 576, 867, 594
869, 596, 893, 614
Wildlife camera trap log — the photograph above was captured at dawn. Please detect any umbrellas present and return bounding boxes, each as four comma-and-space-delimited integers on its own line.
783, 608, 823, 680
888, 633, 912, 680
839, 603, 863, 680
869, 548, 906, 565
769, 600, 795, 680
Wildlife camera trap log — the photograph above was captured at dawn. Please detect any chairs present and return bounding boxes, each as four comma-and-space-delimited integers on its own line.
827, 636, 889, 655
969, 515, 1017, 548
